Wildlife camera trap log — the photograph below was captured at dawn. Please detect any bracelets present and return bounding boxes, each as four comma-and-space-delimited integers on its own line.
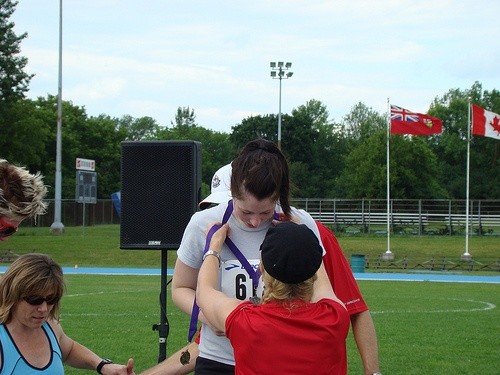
202, 250, 222, 268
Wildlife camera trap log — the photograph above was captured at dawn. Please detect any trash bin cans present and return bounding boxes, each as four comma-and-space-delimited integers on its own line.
351, 255, 365, 273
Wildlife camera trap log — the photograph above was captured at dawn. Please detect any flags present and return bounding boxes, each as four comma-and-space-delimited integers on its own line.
390, 104, 442, 136
472, 104, 500, 140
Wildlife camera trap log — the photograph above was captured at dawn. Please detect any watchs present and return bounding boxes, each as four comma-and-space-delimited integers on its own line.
97, 359, 112, 375
372, 372, 382, 375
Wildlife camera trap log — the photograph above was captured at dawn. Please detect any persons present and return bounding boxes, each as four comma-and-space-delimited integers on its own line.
128, 138, 382, 375
0, 159, 49, 242
0, 252, 134, 375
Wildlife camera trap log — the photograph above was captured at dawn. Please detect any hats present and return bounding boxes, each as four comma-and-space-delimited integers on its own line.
259, 221, 323, 284
198, 163, 233, 210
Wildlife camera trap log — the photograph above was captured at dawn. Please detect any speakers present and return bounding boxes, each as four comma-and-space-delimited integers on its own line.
119, 140, 201, 250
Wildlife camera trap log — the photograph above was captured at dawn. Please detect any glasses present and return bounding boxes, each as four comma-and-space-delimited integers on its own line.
22, 294, 60, 305
0, 218, 18, 238
